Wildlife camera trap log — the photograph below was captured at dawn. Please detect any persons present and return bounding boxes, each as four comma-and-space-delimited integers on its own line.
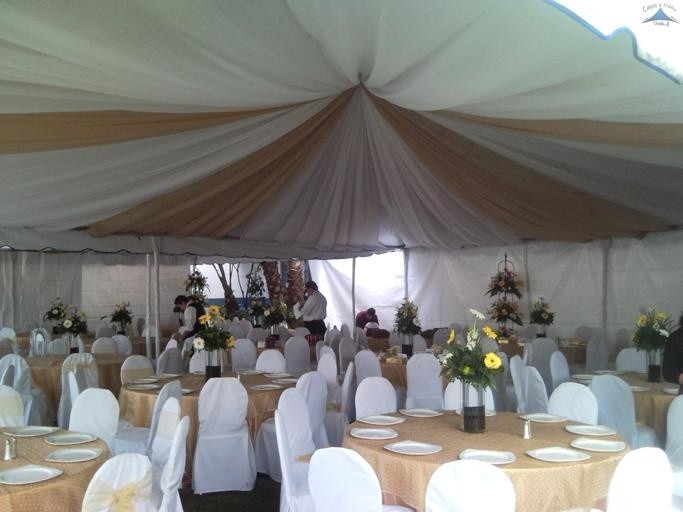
356, 307, 379, 330
174, 295, 207, 338
298, 280, 328, 336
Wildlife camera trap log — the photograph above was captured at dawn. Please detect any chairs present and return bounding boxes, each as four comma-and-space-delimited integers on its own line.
155, 349, 180, 375
325, 363, 359, 438
319, 346, 338, 362
354, 351, 405, 404
617, 347, 648, 382
318, 357, 338, 388
121, 355, 154, 383
146, 415, 192, 512
277, 325, 288, 340
317, 357, 344, 407
2, 327, 22, 357
549, 350, 569, 388
413, 334, 427, 354
47, 338, 68, 357
233, 340, 258, 371
586, 333, 610, 374
284, 334, 315, 377
445, 375, 497, 413
95, 327, 114, 341
435, 330, 448, 351
354, 378, 397, 416
139, 398, 182, 510
388, 334, 403, 353
576, 324, 589, 345
66, 373, 132, 450
248, 328, 267, 347
190, 378, 258, 493
401, 354, 446, 412
188, 345, 226, 374
425, 459, 519, 512
322, 329, 339, 347
520, 368, 550, 414
92, 337, 118, 356
522, 325, 542, 344
113, 378, 182, 468
507, 357, 522, 408
256, 347, 285, 373
526, 339, 558, 390
109, 334, 133, 354
28, 328, 50, 357
82, 453, 154, 512
290, 328, 310, 339
0, 384, 25, 427
615, 327, 631, 352
330, 334, 343, 352
308, 446, 415, 511
336, 338, 349, 382
353, 326, 369, 354
297, 373, 329, 451
312, 340, 323, 369
566, 447, 674, 512
548, 382, 599, 430
233, 320, 253, 338
591, 375, 655, 450
56, 352, 100, 434
3, 355, 42, 430
69, 388, 120, 457
494, 352, 508, 399
255, 387, 310, 483
326, 362, 355, 444
663, 392, 683, 476
481, 337, 497, 363
341, 323, 352, 337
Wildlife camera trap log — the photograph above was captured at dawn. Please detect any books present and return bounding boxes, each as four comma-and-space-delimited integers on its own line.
292, 302, 303, 320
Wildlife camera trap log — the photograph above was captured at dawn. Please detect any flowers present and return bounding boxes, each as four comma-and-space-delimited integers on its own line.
194, 306, 237, 354
53, 309, 90, 336
182, 269, 211, 306
483, 251, 525, 336
531, 297, 556, 326
40, 295, 77, 323
249, 294, 269, 316
389, 297, 422, 335
439, 311, 509, 393
99, 301, 137, 326
263, 301, 293, 328
628, 305, 674, 351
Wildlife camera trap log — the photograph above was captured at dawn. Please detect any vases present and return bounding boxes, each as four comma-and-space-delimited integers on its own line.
534, 324, 547, 339
497, 319, 506, 334
641, 347, 665, 381
271, 322, 280, 336
52, 320, 61, 333
202, 348, 223, 382
118, 330, 127, 336
462, 379, 487, 433
254, 315, 263, 326
401, 335, 413, 355
68, 334, 80, 354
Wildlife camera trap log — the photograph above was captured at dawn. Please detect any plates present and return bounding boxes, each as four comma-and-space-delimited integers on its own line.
273, 378, 299, 384
359, 414, 406, 425
350, 428, 398, 440
571, 438, 625, 453
236, 370, 263, 375
128, 384, 160, 390
0, 464, 62, 485
399, 408, 444, 418
579, 377, 592, 385
460, 449, 515, 464
630, 386, 649, 391
565, 425, 616, 436
44, 433, 95, 445
45, 447, 103, 462
148, 374, 181, 378
663, 388, 678, 395
182, 388, 193, 394
456, 409, 496, 417
520, 413, 568, 423
594, 371, 624, 376
526, 447, 591, 462
5, 426, 61, 436
571, 375, 595, 379
265, 373, 291, 379
127, 378, 158, 384
248, 383, 284, 391
634, 369, 648, 374
384, 440, 442, 456
